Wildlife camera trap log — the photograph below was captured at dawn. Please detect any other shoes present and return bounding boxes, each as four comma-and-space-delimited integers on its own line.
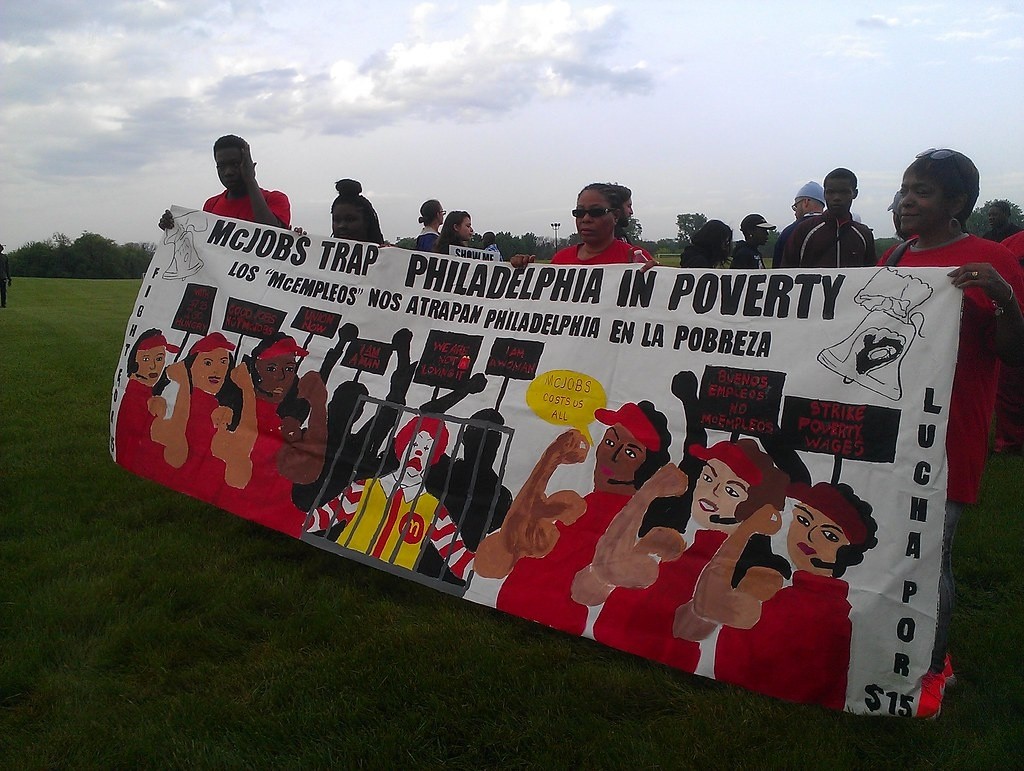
988, 437, 1018, 455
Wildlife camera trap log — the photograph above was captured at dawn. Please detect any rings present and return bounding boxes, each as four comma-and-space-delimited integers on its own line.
971, 271, 978, 279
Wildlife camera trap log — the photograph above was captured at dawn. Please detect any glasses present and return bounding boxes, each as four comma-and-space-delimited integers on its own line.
572, 208, 616, 217
792, 198, 810, 211
439, 211, 446, 216
916, 148, 965, 181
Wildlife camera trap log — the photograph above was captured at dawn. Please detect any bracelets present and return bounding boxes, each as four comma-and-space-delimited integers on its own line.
992, 284, 1013, 315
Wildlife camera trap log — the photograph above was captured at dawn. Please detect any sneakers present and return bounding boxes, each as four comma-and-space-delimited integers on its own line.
914, 668, 945, 720
942, 653, 957, 686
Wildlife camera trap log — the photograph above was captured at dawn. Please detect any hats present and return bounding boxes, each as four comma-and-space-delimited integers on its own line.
740, 214, 776, 231
794, 181, 826, 207
887, 190, 904, 215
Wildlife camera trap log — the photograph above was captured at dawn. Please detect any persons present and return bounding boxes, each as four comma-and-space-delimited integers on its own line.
416, 199, 446, 252
679, 220, 733, 269
430, 210, 474, 255
158, 135, 292, 232
294, 179, 384, 244
876, 148, 1023, 717
482, 232, 503, 261
729, 214, 777, 269
772, 180, 826, 269
509, 183, 657, 272
780, 168, 879, 268
0, 244, 12, 308
